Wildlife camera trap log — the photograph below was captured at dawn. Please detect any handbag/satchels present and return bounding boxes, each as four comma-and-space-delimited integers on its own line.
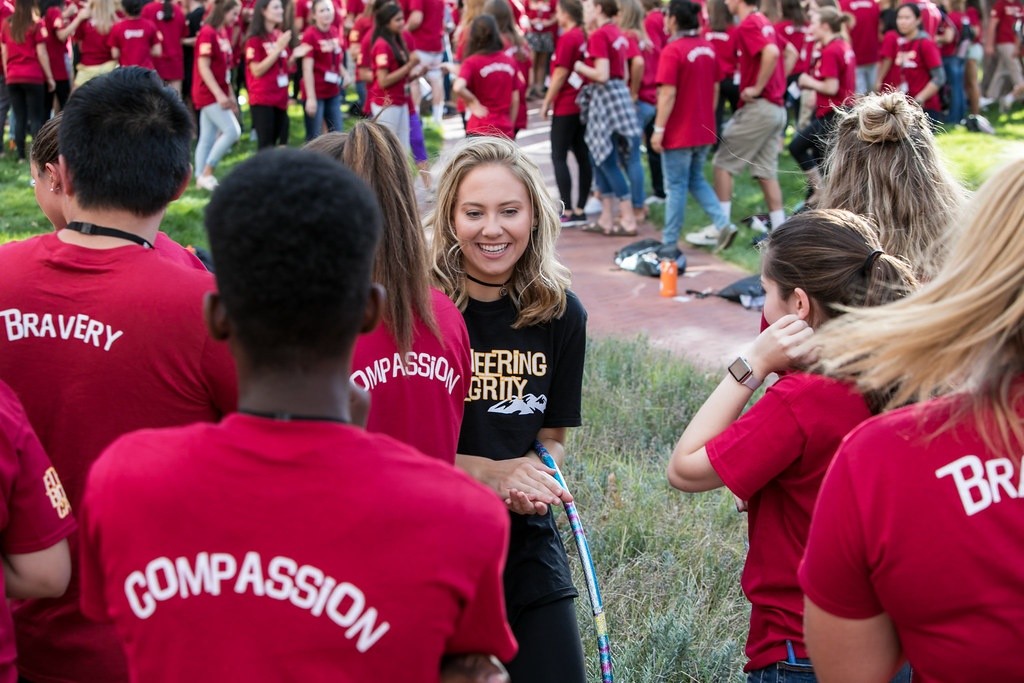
614, 236, 685, 275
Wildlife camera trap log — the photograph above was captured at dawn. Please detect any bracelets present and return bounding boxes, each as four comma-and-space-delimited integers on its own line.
653, 125, 665, 132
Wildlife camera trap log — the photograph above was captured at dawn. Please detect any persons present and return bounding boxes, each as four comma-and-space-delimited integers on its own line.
0, 67, 239, 683
427, 136, 589, 682
0, 0, 1024, 259
30, 111, 206, 271
0, 379, 77, 683
77, 146, 518, 683
760, 90, 972, 335
296, 123, 473, 468
667, 207, 922, 683
795, 154, 1024, 683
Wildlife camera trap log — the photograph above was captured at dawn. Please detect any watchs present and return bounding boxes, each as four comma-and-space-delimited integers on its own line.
727, 357, 764, 390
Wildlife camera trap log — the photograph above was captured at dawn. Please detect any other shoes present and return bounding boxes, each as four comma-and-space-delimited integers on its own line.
196, 174, 220, 191
685, 224, 716, 246
558, 209, 588, 227
584, 197, 603, 215
713, 224, 738, 254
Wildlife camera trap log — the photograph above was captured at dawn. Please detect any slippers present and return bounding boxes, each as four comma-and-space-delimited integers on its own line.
579, 220, 639, 236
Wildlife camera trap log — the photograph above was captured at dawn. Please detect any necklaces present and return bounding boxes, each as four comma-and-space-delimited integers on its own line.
463, 272, 513, 296
233, 407, 348, 424
63, 220, 153, 250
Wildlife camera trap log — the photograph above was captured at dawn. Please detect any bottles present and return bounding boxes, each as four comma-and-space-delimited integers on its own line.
659, 248, 677, 296
